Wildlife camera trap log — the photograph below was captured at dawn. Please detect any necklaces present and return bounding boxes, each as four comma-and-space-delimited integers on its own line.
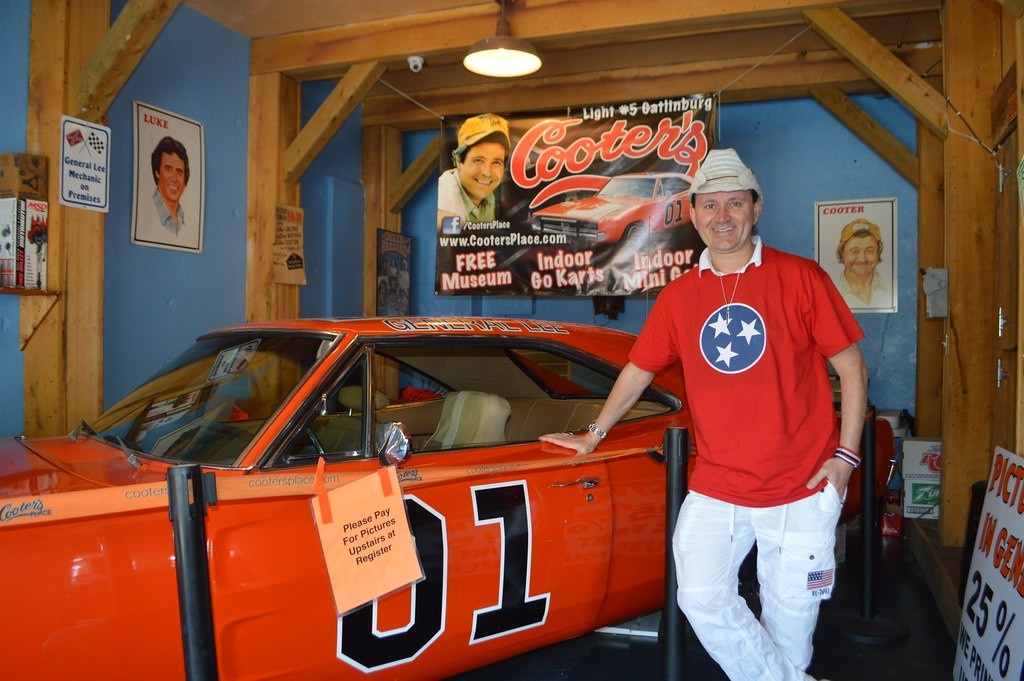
717, 273, 741, 323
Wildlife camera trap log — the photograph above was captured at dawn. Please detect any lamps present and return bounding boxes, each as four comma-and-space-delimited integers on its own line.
462, 0, 542, 78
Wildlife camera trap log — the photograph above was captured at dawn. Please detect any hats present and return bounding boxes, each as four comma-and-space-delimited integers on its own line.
455, 112, 510, 156
687, 148, 764, 203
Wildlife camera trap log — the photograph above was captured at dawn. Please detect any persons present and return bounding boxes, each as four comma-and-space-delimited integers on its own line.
833, 219, 891, 308
437, 113, 511, 232
152, 136, 190, 234
538, 150, 865, 681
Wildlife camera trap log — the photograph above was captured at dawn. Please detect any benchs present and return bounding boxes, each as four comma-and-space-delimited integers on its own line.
504, 398, 670, 446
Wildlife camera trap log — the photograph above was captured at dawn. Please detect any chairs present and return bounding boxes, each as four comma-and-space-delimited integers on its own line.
419, 390, 512, 453
298, 385, 390, 465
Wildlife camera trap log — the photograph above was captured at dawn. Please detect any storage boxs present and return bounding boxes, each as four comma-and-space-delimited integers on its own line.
0, 198, 47, 290
898, 437, 941, 520
893, 422, 909, 437
881, 492, 903, 537
874, 409, 901, 429
0, 151, 47, 201
886, 429, 912, 491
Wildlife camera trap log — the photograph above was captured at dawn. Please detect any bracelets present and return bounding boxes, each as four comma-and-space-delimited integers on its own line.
834, 446, 862, 469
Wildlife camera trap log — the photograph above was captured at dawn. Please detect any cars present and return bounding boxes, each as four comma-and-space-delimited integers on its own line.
1, 316, 901, 681
538, 171, 703, 245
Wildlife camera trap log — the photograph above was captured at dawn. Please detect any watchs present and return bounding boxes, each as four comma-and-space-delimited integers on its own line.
588, 419, 607, 440
